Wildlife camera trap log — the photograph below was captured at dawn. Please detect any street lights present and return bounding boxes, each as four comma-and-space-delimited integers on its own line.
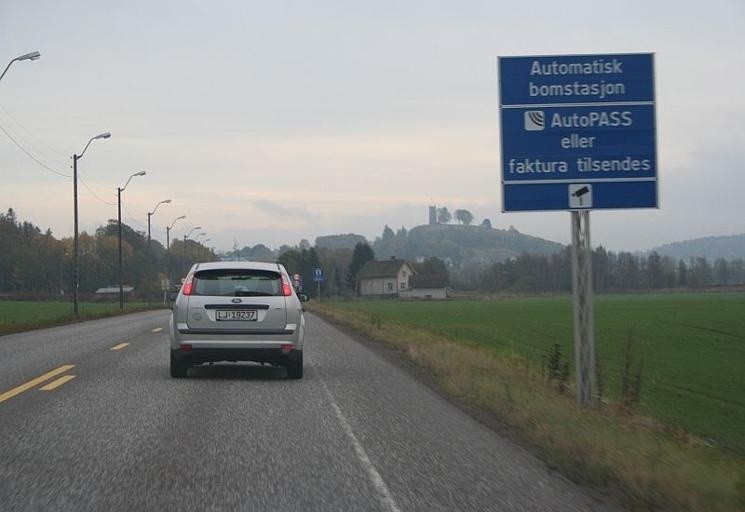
148, 200, 210, 269
1, 51, 40, 80
118, 171, 145, 309
73, 131, 110, 312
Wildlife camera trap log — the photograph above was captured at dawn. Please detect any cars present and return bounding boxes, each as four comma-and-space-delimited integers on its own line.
168, 262, 308, 379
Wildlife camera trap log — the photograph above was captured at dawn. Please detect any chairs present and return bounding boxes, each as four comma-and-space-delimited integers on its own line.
203, 276, 220, 295
256, 279, 274, 296
